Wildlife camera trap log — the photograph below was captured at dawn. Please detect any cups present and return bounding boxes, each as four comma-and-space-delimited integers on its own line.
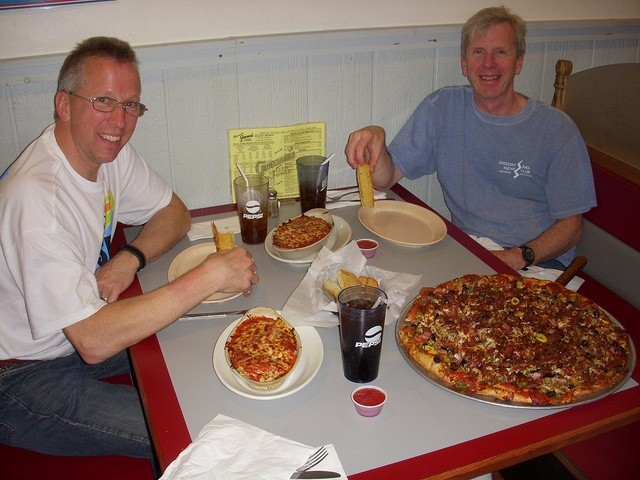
232, 174, 269, 245
296, 155, 330, 214
337, 284, 388, 384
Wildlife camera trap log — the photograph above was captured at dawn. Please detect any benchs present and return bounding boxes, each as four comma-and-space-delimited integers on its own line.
552, 59, 640, 478
0, 368, 156, 479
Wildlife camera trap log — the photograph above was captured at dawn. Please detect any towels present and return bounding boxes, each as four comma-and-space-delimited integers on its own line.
326, 188, 387, 209
157, 413, 349, 480
469, 234, 586, 292
187, 216, 241, 241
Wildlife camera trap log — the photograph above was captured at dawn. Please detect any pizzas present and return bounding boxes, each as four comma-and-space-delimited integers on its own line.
225, 316, 297, 385
272, 210, 331, 250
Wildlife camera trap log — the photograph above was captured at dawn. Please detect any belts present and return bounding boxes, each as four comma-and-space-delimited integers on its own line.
0, 359, 29, 367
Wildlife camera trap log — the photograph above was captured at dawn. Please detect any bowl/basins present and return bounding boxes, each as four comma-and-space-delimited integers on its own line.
271, 207, 334, 260
224, 307, 303, 392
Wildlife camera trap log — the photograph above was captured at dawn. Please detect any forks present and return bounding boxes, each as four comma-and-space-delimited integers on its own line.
326, 191, 360, 201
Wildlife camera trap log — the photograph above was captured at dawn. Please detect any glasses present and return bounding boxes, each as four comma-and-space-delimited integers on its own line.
69, 91, 148, 118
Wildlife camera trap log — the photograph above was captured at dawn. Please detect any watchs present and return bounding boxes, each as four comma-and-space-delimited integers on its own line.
518, 244, 535, 266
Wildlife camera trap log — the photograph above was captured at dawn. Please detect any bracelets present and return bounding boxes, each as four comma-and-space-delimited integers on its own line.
120, 243, 145, 272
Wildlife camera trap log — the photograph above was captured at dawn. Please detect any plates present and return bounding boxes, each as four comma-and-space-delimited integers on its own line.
212, 310, 326, 402
358, 200, 448, 249
264, 215, 352, 267
167, 241, 245, 305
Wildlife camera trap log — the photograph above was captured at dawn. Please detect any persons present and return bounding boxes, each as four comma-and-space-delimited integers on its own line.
2, 36, 258, 461
345, 6, 597, 271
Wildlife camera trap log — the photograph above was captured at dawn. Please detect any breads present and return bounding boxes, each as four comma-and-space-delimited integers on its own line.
323, 267, 378, 306
357, 162, 377, 209
212, 221, 236, 252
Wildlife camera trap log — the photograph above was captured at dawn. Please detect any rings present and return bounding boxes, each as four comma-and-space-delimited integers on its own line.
100, 297, 108, 301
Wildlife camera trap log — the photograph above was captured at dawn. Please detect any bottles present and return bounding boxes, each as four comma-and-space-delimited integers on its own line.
268, 190, 280, 217
